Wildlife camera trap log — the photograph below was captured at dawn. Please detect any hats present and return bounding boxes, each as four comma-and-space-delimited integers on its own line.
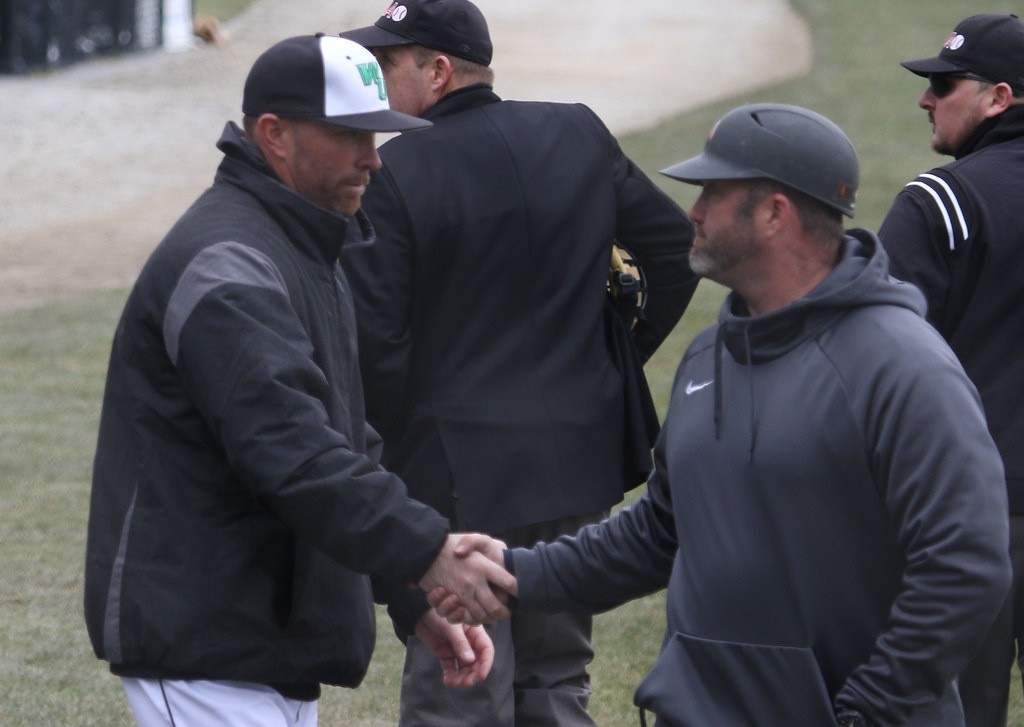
339, 0, 494, 67
241, 32, 435, 133
900, 13, 1023, 77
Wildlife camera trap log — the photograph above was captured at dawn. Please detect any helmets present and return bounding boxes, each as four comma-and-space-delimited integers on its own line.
658, 102, 860, 220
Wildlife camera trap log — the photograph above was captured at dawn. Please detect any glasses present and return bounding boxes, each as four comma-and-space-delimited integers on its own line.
930, 71, 993, 98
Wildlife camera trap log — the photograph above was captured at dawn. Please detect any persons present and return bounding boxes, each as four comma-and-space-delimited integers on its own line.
877, 11, 1024, 691
426, 104, 1014, 727
84, 32, 521, 726
336, 0, 701, 726
